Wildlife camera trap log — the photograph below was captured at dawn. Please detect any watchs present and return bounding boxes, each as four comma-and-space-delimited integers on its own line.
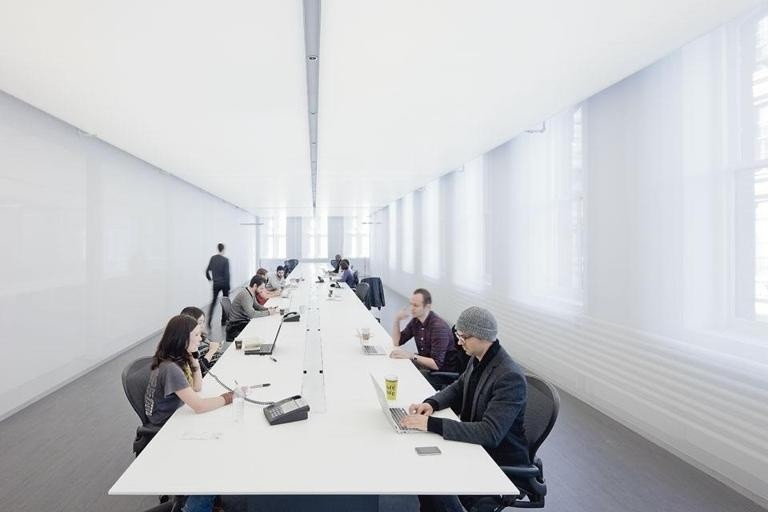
413, 352, 418, 361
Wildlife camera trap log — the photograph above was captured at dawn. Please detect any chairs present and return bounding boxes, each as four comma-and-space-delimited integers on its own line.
120, 356, 162, 459
352, 271, 383, 307
495, 374, 562, 510
431, 324, 471, 386
220, 297, 232, 320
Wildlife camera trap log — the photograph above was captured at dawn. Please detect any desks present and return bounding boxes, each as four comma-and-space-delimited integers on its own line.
108, 261, 521, 512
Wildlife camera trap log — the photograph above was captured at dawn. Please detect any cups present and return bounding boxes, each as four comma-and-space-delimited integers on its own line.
235, 337, 242, 350
234, 393, 244, 421
363, 327, 369, 346
385, 373, 398, 401
280, 308, 284, 315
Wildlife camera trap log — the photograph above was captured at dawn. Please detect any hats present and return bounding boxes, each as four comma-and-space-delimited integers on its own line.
455, 305, 499, 342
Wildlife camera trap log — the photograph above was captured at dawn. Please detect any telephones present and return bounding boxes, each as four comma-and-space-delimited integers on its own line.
283, 312, 300, 322
192, 351, 199, 359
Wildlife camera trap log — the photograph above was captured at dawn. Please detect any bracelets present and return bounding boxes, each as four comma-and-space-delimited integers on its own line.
220, 391, 234, 406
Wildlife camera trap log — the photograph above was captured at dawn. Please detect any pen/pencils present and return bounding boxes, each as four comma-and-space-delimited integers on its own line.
270, 356, 277, 362
250, 384, 270, 388
234, 380, 240, 387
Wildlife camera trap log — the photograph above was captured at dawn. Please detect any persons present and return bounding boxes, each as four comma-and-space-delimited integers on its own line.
399, 305, 530, 512
180, 306, 225, 376
143, 314, 248, 511
266, 266, 285, 291
390, 288, 458, 383
255, 268, 280, 305
205, 243, 230, 328
328, 254, 355, 289
229, 274, 280, 321
